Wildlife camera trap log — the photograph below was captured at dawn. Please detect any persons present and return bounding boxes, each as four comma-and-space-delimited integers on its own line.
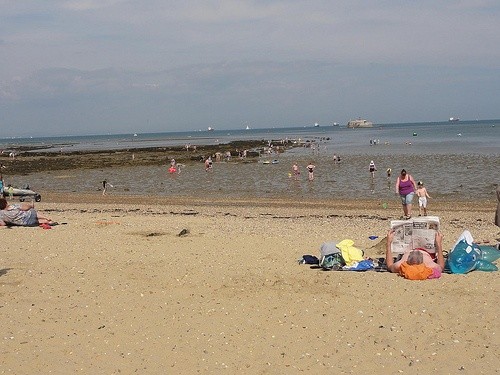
0, 198, 58, 226
6, 184, 15, 200
0, 147, 17, 158
61, 147, 63, 155
416, 181, 434, 215
369, 135, 412, 146
170, 136, 340, 181
385, 229, 444, 273
387, 168, 392, 184
102, 179, 111, 196
395, 168, 417, 219
369, 160, 378, 180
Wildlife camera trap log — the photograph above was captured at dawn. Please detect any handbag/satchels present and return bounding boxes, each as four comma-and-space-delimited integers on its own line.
319, 241, 341, 267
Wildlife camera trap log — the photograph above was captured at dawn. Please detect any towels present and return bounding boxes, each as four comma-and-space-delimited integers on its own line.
334, 240, 454, 274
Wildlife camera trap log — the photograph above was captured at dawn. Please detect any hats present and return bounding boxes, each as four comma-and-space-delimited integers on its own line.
418, 181, 423, 185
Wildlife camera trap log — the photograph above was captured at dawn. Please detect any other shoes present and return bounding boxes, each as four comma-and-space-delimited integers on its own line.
424, 213, 427, 216
419, 215, 421, 216
406, 215, 411, 219
